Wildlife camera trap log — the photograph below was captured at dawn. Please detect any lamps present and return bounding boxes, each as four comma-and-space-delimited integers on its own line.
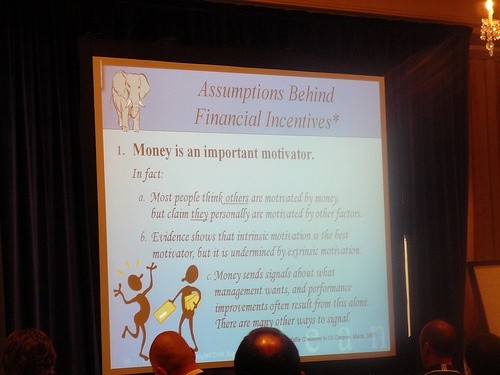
480, 0, 500, 56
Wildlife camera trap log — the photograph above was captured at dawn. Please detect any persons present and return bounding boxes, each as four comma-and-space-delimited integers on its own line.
149, 331, 207, 375
234, 327, 304, 375
419, 320, 461, 375
463, 330, 500, 375
0, 328, 56, 375
